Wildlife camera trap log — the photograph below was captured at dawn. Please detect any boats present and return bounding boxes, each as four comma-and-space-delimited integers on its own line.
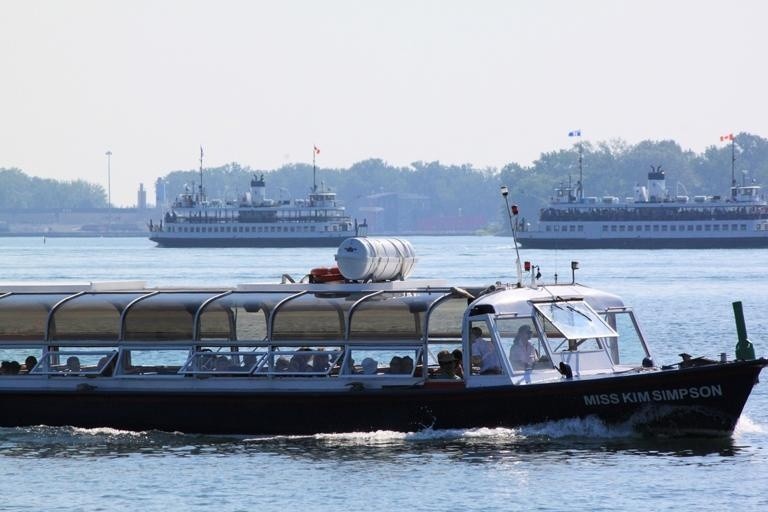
0, 239, 767, 449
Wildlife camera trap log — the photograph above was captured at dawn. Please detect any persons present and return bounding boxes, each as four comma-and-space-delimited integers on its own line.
202, 352, 218, 371
66, 356, 81, 377
470, 327, 504, 376
388, 356, 405, 374
109, 350, 146, 374
312, 351, 331, 374
0, 361, 11, 375
275, 356, 289, 371
508, 324, 540, 372
301, 347, 313, 362
97, 357, 112, 377
427, 349, 461, 380
215, 355, 229, 372
7, 360, 22, 375
25, 356, 38, 371
241, 354, 258, 372
449, 348, 464, 379
288, 349, 313, 371
401, 355, 414, 372
360, 357, 378, 374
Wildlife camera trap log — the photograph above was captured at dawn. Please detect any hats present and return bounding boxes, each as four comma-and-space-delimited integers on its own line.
390, 356, 407, 373
438, 350, 458, 362
294, 348, 313, 360
362, 358, 378, 375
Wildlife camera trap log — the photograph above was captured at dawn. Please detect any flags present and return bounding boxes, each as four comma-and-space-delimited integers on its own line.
720, 134, 734, 141
314, 145, 321, 155
568, 130, 581, 137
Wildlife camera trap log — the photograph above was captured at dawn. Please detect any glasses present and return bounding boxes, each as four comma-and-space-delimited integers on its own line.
527, 331, 533, 334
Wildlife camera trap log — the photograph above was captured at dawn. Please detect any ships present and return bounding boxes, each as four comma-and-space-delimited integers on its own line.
147, 145, 368, 249
512, 130, 766, 251
